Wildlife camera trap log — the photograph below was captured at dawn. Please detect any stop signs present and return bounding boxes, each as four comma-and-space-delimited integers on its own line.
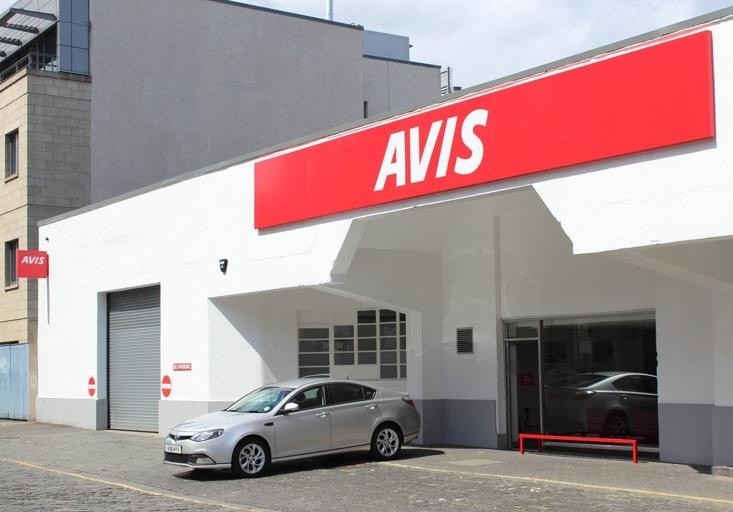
87, 376, 96, 396
161, 375, 172, 397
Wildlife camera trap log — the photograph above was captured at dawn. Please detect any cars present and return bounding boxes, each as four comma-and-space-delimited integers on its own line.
543, 372, 657, 447
165, 376, 420, 480
